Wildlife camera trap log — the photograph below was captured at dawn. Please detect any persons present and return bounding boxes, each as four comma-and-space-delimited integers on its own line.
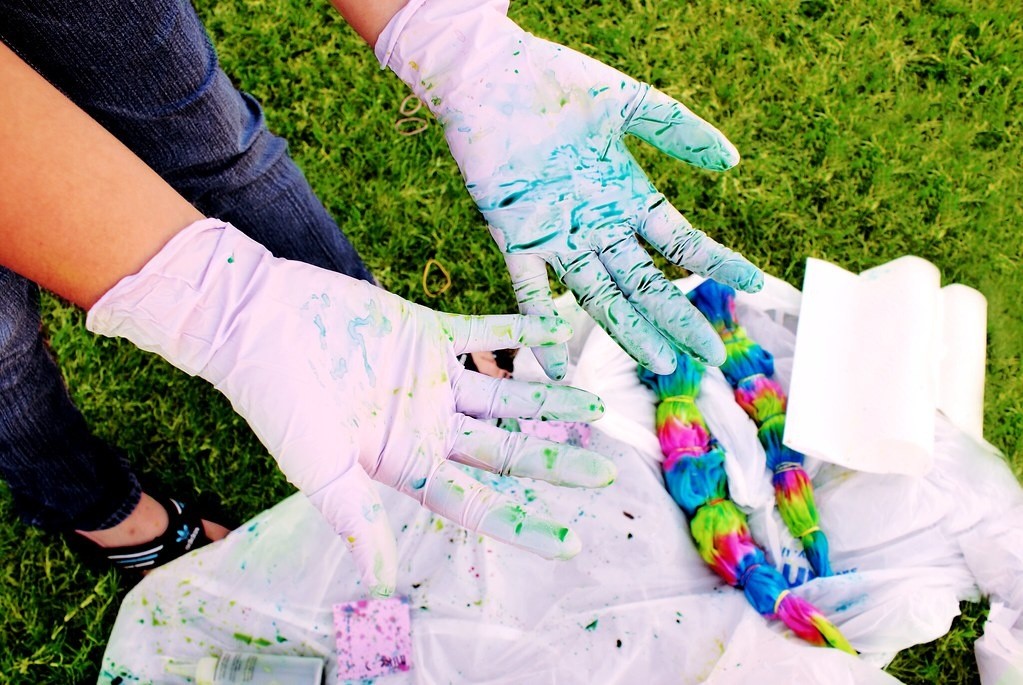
0, 0, 762, 590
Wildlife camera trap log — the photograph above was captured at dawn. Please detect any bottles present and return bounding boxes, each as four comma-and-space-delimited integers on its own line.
166, 651, 324, 685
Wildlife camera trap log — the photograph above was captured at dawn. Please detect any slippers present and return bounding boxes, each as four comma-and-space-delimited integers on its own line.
63, 495, 237, 589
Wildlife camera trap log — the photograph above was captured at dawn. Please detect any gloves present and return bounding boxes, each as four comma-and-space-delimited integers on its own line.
375, 0, 765, 380
86, 217, 617, 600
